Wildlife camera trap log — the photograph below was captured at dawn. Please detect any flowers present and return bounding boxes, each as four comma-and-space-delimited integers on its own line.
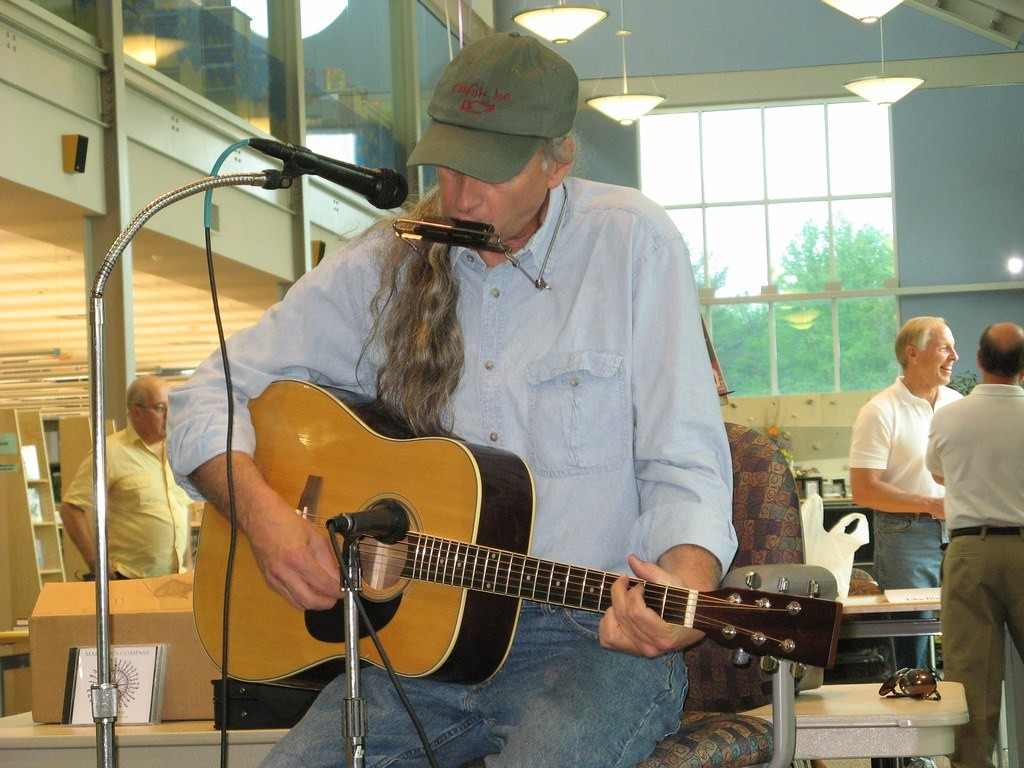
751, 395, 799, 465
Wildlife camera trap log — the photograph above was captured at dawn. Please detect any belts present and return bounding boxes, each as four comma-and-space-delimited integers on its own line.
949, 526, 1021, 537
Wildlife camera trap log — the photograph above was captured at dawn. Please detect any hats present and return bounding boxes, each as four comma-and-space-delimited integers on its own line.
405, 31, 579, 183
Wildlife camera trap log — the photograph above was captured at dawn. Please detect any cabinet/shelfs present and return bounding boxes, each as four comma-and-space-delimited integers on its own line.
206, 5, 255, 121
0, 409, 67, 618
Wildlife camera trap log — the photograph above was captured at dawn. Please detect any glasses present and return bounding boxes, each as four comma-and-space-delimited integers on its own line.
133, 400, 167, 412
879, 668, 941, 701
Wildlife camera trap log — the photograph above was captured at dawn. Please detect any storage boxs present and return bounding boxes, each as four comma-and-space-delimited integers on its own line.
28, 572, 221, 722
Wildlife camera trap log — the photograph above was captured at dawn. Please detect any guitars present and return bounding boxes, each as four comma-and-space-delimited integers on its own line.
191, 381, 844, 688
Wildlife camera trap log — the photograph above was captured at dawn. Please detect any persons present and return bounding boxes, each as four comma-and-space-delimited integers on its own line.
849, 317, 966, 768
165, 30, 739, 768
924, 320, 1024, 768
60, 375, 195, 582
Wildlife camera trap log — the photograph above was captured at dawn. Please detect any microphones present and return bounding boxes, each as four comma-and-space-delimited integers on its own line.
324, 498, 408, 545
247, 139, 408, 210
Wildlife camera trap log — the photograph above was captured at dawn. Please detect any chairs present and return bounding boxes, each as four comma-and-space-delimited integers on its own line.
484, 424, 839, 768
837, 568, 900, 678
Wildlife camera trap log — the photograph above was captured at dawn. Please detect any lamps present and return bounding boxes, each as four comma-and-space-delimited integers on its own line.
842, 17, 926, 108
586, 0, 667, 126
511, 0, 610, 44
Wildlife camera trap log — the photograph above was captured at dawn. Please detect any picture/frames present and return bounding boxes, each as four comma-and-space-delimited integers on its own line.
795, 476, 846, 499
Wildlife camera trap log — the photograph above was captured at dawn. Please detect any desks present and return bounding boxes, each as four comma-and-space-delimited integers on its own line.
842, 586, 941, 681
735, 680, 969, 768
